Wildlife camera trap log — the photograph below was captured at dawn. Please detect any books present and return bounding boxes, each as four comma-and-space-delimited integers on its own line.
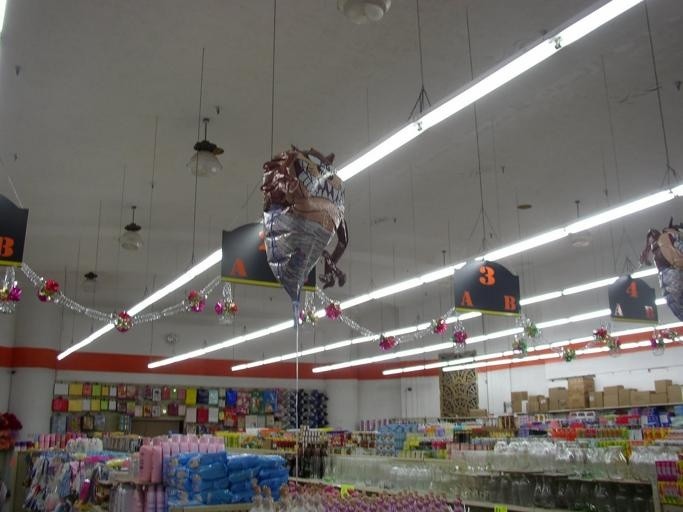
511, 375, 682, 413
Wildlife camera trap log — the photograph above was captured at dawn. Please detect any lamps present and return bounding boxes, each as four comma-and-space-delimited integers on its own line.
76, 117, 227, 293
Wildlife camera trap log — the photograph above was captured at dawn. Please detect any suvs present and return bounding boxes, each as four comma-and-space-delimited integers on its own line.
64, 433, 104, 454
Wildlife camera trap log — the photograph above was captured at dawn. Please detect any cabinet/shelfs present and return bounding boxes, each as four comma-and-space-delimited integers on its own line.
0, 406, 682, 511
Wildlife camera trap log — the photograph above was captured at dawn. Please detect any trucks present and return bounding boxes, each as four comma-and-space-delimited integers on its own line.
258, 144, 349, 309
639, 216, 683, 322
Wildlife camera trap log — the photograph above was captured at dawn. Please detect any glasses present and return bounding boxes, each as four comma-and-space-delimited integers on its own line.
76, 117, 227, 293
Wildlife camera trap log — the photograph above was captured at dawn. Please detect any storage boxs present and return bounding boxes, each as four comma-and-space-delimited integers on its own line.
511, 375, 682, 413
